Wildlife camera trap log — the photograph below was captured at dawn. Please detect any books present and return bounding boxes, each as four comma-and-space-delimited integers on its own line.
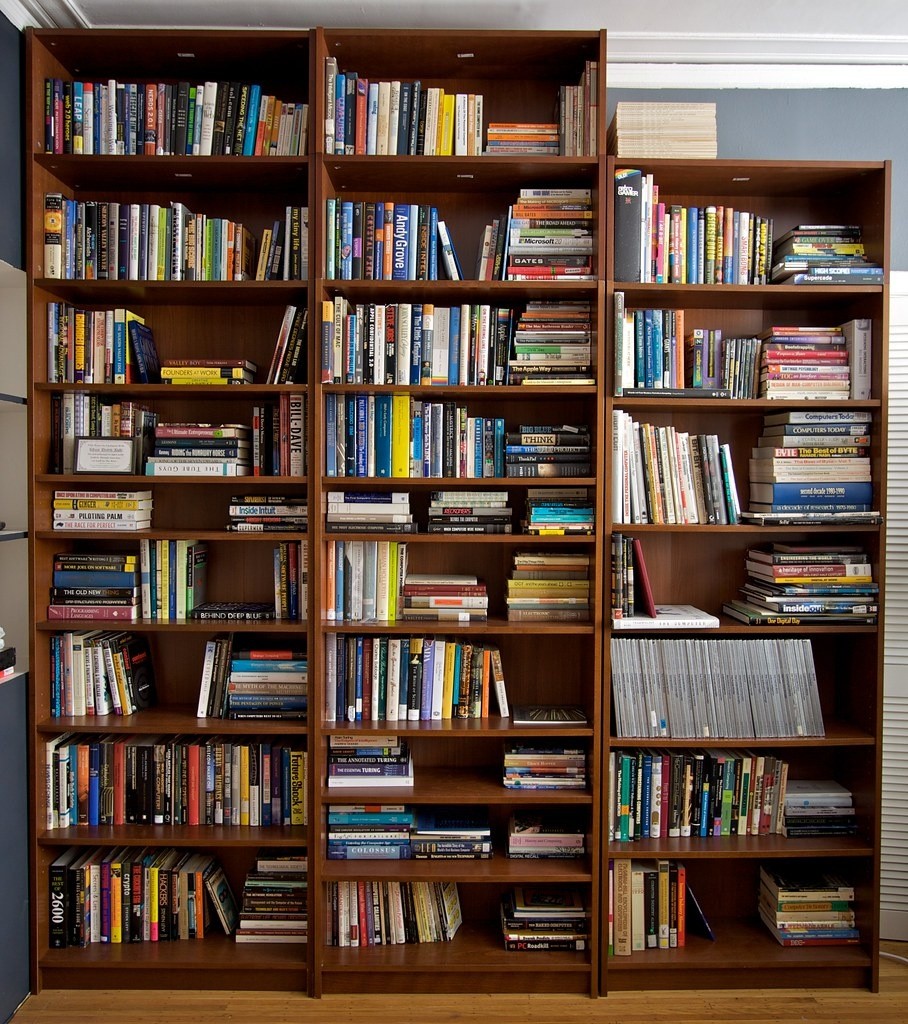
43, 57, 880, 955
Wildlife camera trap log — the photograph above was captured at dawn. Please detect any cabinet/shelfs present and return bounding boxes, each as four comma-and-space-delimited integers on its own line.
312, 25, 611, 999
603, 154, 894, 1002
22, 25, 317, 996
